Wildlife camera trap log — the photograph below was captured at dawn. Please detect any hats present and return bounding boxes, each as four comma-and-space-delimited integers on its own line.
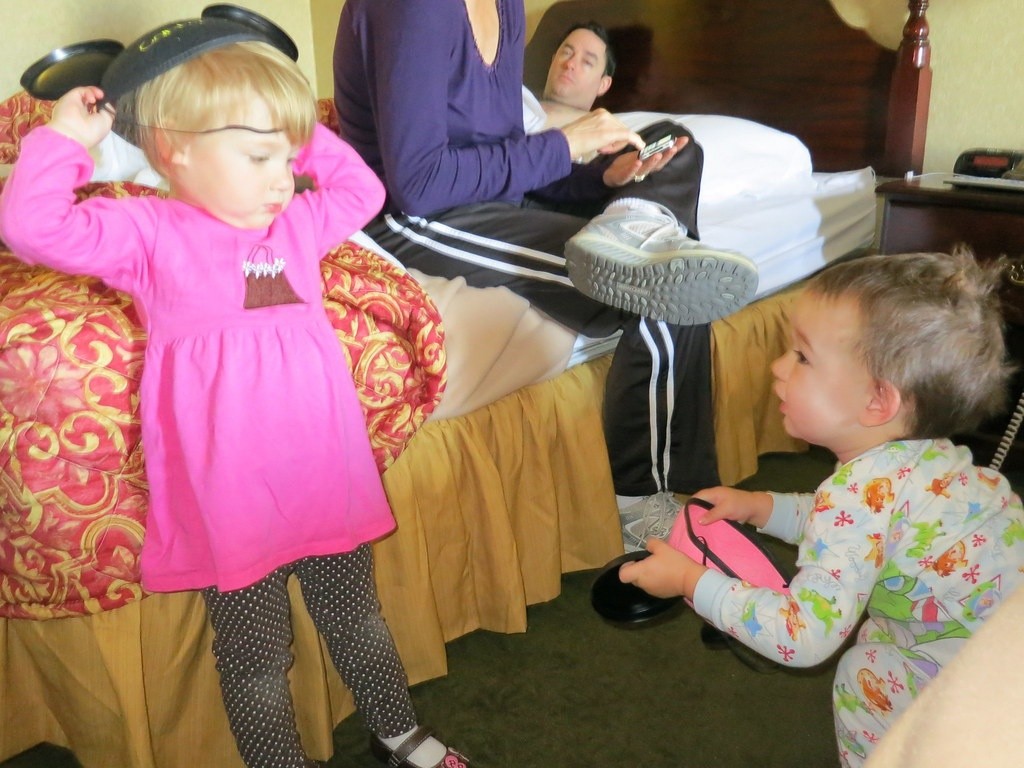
21, 3, 298, 113
591, 500, 792, 675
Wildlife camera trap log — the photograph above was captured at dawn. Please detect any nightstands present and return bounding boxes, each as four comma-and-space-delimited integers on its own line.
873, 172, 1024, 471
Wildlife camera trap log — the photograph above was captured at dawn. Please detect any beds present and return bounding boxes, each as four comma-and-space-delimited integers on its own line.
0, 0, 934, 768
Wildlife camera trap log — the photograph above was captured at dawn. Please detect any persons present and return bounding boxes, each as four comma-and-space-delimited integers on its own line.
333, 1, 754, 556
621, 247, 1024, 768
0, 42, 480, 768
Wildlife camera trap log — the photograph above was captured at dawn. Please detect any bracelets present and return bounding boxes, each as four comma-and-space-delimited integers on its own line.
567, 139, 584, 165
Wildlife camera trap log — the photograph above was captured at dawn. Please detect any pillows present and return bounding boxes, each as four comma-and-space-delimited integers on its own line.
612, 113, 817, 227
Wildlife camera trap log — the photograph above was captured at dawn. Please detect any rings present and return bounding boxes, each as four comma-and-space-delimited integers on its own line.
634, 173, 648, 183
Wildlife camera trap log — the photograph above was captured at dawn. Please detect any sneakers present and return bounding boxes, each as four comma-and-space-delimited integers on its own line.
617, 491, 687, 553
565, 201, 758, 325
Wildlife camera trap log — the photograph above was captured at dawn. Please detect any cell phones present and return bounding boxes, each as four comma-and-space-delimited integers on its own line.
638, 132, 676, 160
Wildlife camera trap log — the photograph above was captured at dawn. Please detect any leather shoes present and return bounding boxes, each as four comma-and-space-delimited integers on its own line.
371, 726, 471, 768
